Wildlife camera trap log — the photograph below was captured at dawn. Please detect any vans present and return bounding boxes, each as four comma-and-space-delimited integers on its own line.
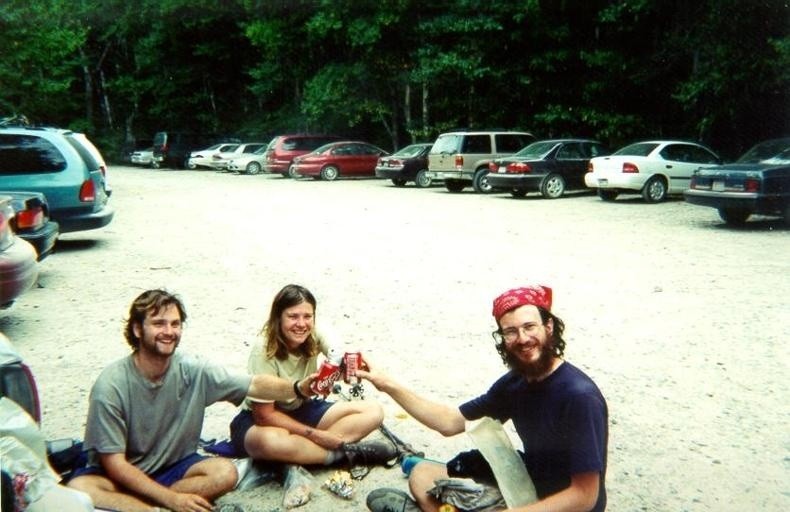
265, 134, 353, 178
153, 130, 209, 169
426, 129, 539, 193
0, 125, 116, 234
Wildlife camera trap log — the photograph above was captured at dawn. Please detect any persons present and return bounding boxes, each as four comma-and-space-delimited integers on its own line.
65, 290, 319, 512
354, 284, 608, 512
229, 285, 396, 485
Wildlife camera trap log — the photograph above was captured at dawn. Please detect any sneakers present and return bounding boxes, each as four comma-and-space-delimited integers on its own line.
339, 441, 398, 468
367, 488, 421, 512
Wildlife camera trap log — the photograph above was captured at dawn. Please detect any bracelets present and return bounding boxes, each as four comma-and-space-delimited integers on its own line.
294, 381, 307, 400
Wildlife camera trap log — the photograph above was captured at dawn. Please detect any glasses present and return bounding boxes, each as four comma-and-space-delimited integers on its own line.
500, 323, 542, 340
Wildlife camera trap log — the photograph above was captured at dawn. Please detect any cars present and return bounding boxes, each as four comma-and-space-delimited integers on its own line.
684, 138, 789, 231
374, 142, 435, 188
189, 143, 224, 172
0, 210, 39, 310
488, 138, 610, 200
292, 141, 391, 181
226, 145, 269, 175
169, 139, 209, 170
0, 190, 60, 264
584, 140, 729, 205
131, 147, 156, 167
210, 142, 266, 168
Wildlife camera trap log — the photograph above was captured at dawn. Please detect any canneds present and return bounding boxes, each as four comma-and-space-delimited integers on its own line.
327, 346, 343, 367
345, 351, 362, 384
310, 360, 341, 395
